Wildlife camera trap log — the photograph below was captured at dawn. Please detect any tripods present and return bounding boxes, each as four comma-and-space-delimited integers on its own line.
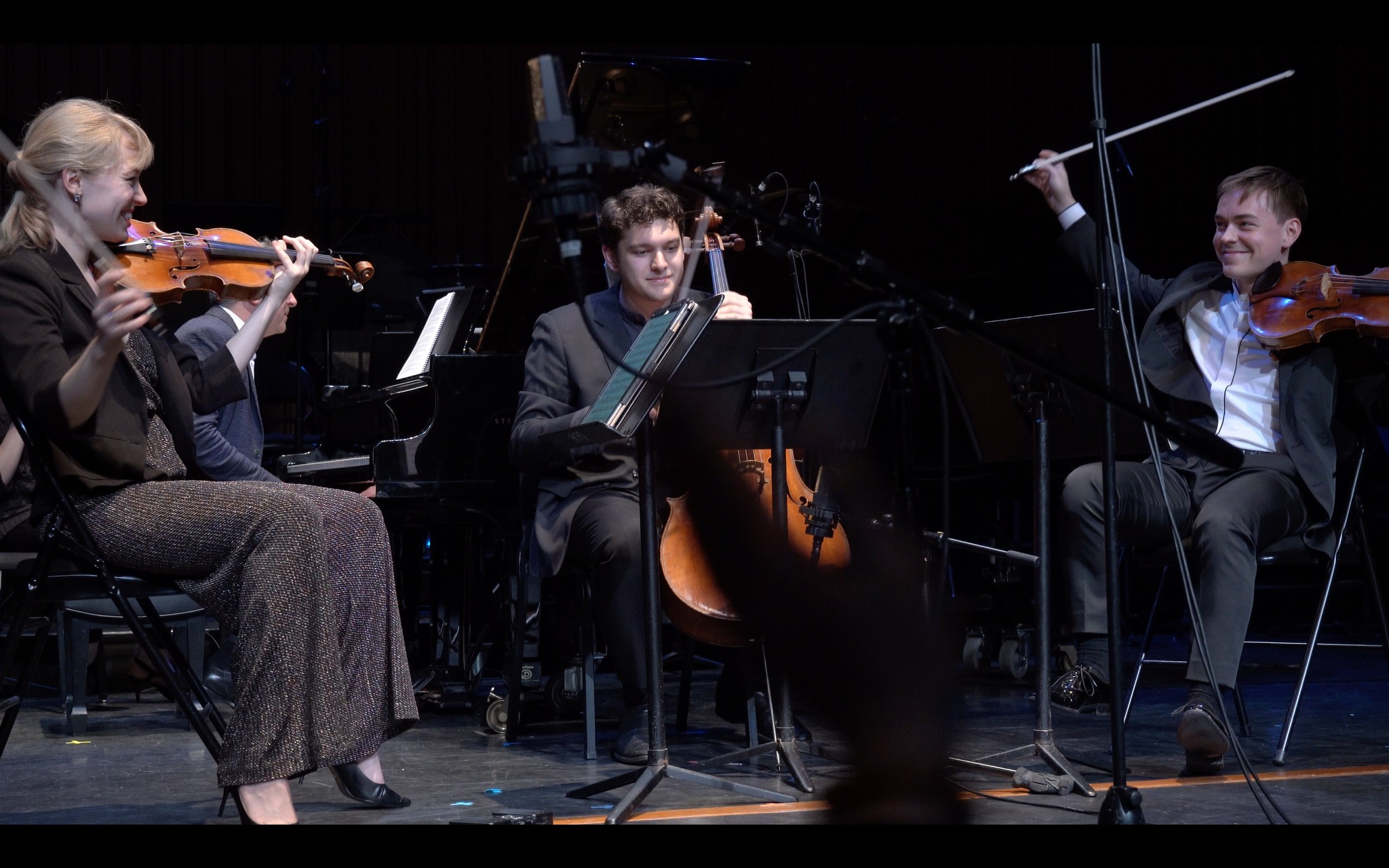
537, 294, 903, 825
931, 307, 1146, 798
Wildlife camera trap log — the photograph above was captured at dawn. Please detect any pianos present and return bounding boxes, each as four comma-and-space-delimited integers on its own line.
275, 351, 522, 727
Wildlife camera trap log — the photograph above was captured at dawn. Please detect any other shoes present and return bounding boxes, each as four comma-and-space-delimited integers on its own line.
202, 657, 236, 709
610, 710, 652, 763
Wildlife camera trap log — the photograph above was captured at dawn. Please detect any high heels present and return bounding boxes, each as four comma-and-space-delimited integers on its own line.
299, 762, 412, 808
217, 783, 302, 826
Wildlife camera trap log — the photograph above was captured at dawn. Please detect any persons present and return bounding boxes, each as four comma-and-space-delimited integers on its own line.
0, 96, 424, 826
1018, 151, 1338, 773
172, 286, 380, 702
506, 184, 822, 765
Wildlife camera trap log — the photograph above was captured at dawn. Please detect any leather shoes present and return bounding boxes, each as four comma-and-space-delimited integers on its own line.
1170, 700, 1229, 774
1050, 662, 1112, 716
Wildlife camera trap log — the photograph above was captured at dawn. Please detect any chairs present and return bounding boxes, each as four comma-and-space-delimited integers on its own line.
1121, 428, 1389, 765
0, 393, 226, 774
522, 452, 760, 764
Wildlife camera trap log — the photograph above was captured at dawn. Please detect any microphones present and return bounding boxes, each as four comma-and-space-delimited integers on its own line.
528, 54, 587, 281
806, 183, 817, 228
722, 173, 772, 235
807, 464, 844, 569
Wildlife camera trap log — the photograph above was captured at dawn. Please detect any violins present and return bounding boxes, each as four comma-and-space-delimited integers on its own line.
89, 214, 372, 308
1246, 260, 1389, 352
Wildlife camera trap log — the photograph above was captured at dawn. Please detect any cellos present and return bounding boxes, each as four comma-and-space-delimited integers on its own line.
655, 200, 850, 776
